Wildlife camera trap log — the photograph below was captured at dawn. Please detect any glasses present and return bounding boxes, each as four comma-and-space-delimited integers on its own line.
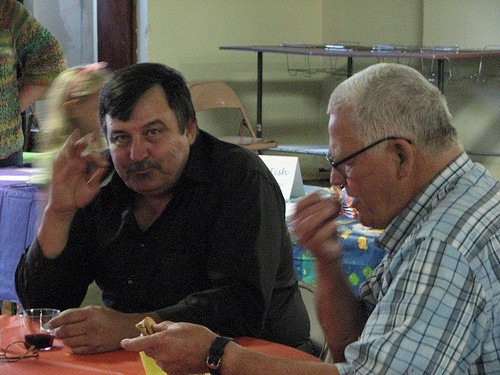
0, 341, 39, 362
327, 136, 412, 179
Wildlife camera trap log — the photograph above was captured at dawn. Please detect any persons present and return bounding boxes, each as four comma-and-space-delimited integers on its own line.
12, 61, 311, 356
37, 62, 119, 166
0, 0, 65, 172
119, 61, 500, 375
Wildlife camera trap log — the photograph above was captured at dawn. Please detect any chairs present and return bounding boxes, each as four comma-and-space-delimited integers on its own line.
189, 82, 277, 155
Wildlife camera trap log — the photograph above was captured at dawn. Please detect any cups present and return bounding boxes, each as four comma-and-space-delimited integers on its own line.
16, 308, 60, 350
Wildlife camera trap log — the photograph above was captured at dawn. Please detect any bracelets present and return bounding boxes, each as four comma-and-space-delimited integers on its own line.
205, 335, 237, 375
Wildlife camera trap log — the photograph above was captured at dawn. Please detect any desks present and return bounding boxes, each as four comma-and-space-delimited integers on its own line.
0, 166, 388, 315
219, 44, 500, 155
267, 143, 329, 157
0, 314, 323, 375
